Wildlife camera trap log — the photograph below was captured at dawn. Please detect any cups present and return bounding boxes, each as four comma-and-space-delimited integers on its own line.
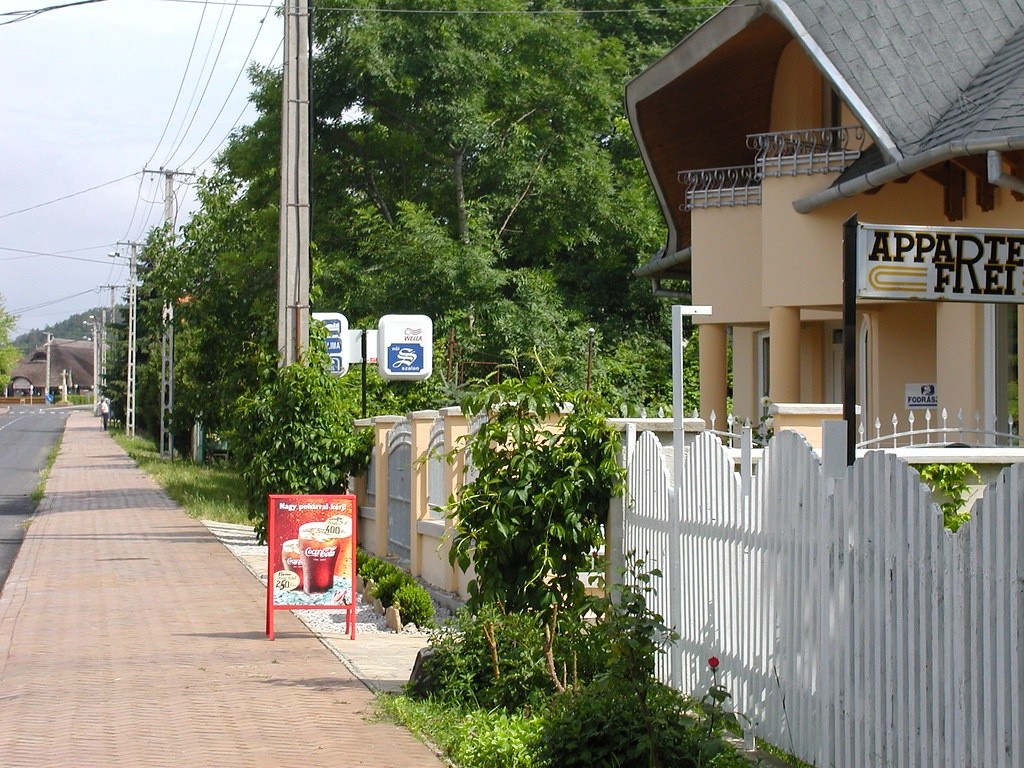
281, 515, 352, 595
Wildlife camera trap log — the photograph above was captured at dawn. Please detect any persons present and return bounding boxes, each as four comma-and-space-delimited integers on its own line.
101, 398, 109, 431
